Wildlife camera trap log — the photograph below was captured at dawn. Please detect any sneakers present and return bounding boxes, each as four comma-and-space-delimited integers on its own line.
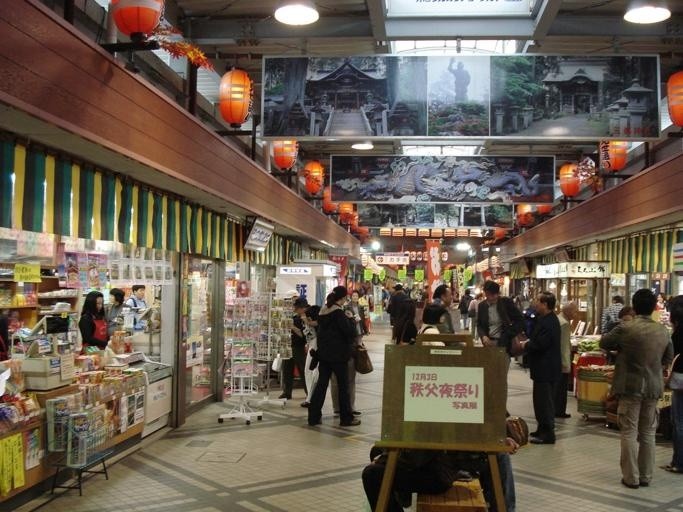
300, 401, 311, 408
339, 417, 362, 426
278, 392, 292, 400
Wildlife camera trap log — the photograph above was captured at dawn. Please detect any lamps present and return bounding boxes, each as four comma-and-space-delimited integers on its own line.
620, 2, 671, 25
275, 1, 320, 28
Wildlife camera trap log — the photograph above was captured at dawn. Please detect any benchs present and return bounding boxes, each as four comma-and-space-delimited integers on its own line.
410, 470, 488, 512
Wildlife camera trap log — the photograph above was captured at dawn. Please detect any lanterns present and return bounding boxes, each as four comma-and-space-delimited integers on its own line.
338, 203, 353, 222
517, 204, 532, 227
560, 162, 579, 199
303, 159, 325, 195
110, 0, 163, 36
323, 185, 337, 215
219, 70, 255, 128
495, 229, 505, 239
350, 212, 358, 232
536, 204, 552, 215
358, 226, 369, 240
599, 141, 626, 173
667, 71, 683, 132
273, 141, 299, 170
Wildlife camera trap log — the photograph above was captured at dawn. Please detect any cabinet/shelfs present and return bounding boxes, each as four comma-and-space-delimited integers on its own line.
34, 271, 79, 316
215, 297, 265, 426
258, 298, 293, 409
43, 392, 116, 496
0, 261, 36, 332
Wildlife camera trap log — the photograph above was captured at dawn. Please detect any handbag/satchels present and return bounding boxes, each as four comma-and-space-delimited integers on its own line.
506, 410, 529, 448
355, 342, 374, 375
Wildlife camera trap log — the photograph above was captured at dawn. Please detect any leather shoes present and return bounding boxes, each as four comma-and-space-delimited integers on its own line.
529, 436, 556, 444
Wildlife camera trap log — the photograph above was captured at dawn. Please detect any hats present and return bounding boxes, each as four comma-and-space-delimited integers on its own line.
292, 297, 310, 307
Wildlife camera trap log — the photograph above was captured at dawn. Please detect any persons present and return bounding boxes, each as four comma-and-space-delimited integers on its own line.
432, 284, 455, 334
331, 306, 362, 417
520, 291, 561, 444
601, 296, 624, 337
125, 285, 148, 332
301, 305, 321, 408
522, 298, 534, 380
553, 300, 579, 418
448, 437, 518, 512
278, 290, 308, 399
0, 308, 8, 361
448, 57, 470, 102
108, 288, 133, 337
79, 291, 109, 355
618, 307, 636, 320
600, 288, 675, 489
387, 284, 407, 339
478, 280, 523, 417
655, 293, 667, 311
417, 303, 448, 346
361, 446, 454, 512
457, 289, 473, 330
468, 293, 483, 344
293, 298, 308, 353
346, 290, 369, 347
405, 286, 428, 301
308, 285, 362, 426
658, 295, 683, 473
392, 299, 418, 345
